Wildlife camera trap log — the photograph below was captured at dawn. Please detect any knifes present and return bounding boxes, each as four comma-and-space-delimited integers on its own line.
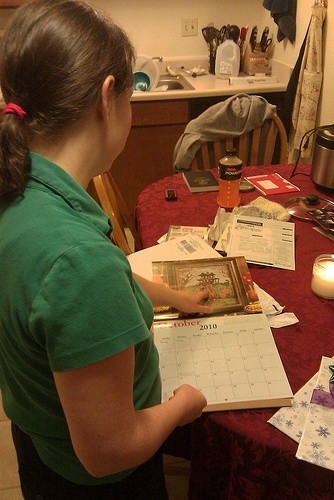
249, 26, 270, 53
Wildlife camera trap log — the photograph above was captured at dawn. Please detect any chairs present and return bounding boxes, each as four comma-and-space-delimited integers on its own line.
174, 98, 290, 163
92, 168, 137, 257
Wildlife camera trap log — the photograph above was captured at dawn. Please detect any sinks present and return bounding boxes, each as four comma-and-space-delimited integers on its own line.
156, 72, 196, 91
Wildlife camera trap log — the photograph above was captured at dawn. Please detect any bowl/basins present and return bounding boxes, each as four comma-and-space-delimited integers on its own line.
132, 52, 161, 93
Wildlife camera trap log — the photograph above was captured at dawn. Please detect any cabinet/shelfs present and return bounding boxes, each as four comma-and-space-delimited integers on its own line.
77, 59, 292, 230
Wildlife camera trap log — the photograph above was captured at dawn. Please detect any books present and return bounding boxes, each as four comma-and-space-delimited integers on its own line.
183, 171, 219, 193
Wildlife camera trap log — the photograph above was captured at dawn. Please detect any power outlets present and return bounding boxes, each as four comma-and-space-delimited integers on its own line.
182, 17, 200, 41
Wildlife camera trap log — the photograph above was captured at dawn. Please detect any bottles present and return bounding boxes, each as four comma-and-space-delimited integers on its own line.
215, 34, 241, 80
217, 149, 243, 208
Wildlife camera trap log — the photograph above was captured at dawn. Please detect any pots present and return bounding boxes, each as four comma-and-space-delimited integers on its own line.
310, 124, 334, 193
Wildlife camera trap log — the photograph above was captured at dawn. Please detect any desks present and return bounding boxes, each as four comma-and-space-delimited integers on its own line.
129, 150, 334, 499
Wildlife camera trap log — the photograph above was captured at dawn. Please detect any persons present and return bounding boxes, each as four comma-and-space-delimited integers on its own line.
0, 0, 212, 500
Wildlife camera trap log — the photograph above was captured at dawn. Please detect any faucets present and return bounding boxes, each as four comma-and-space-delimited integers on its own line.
139, 56, 163, 72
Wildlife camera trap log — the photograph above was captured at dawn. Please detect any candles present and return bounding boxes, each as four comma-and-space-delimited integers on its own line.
311, 251, 334, 301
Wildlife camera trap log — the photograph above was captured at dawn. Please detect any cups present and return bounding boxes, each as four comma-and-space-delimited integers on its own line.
310, 253, 334, 299
